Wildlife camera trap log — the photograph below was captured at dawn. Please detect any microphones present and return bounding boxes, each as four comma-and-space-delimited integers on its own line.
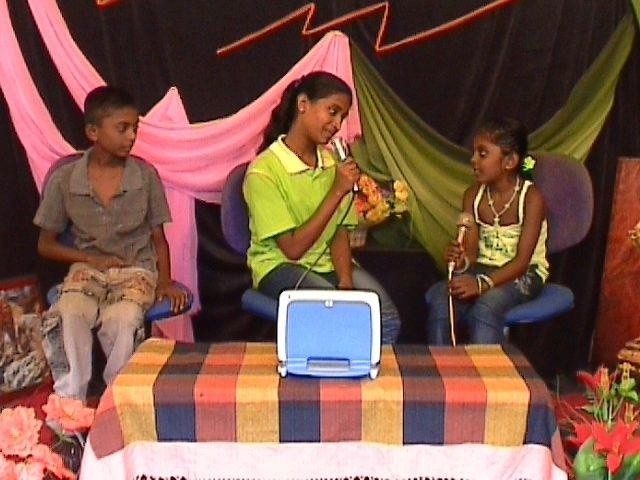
447, 210, 475, 274
329, 135, 358, 192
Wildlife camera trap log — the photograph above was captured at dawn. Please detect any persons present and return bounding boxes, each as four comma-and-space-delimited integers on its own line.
424, 114, 552, 347
242, 67, 404, 348
32, 84, 189, 402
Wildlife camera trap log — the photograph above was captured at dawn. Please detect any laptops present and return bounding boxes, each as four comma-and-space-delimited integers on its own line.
277, 287, 381, 379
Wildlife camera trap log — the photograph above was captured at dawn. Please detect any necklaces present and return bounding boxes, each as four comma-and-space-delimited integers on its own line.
486, 175, 521, 227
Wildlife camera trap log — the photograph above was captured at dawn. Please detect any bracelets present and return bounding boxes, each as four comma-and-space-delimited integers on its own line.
476, 275, 482, 296
479, 273, 494, 290
453, 256, 471, 275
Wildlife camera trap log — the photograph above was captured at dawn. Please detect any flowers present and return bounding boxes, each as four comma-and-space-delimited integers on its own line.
550, 362, 640, 480
1, 390, 94, 478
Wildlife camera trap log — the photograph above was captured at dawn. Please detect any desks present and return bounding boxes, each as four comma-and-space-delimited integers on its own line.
107, 342, 554, 480
